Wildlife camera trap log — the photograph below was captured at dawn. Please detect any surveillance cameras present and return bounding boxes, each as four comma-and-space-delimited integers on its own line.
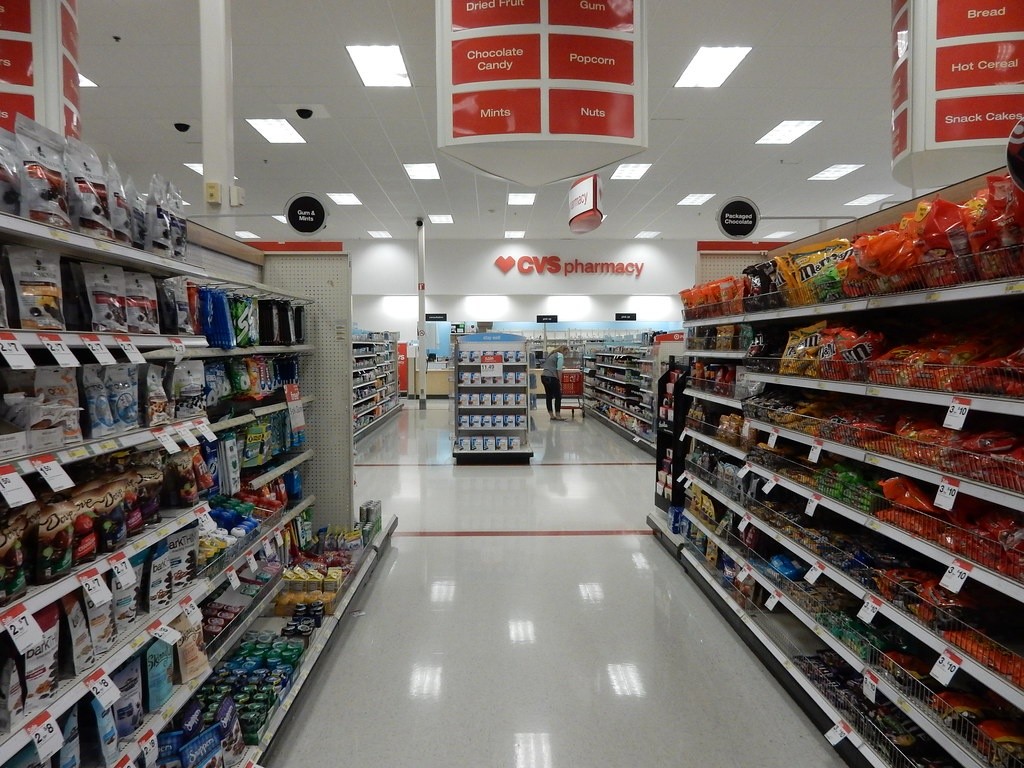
175, 123, 190, 132
296, 109, 313, 119
417, 221, 423, 226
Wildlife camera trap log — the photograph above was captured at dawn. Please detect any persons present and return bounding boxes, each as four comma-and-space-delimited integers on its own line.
541, 345, 569, 421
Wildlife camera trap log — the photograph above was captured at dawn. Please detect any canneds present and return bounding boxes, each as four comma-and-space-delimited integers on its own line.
191, 595, 324, 746
354, 499, 383, 545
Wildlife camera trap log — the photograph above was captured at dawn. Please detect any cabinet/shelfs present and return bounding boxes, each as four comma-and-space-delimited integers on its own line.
740, 243, 1024, 768
582, 353, 657, 450
0, 211, 214, 768
679, 297, 746, 636
452, 362, 534, 466
352, 340, 398, 438
129, 268, 316, 671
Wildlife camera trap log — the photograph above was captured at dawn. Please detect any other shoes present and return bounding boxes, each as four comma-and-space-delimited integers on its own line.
550, 416, 556, 420
556, 416, 567, 420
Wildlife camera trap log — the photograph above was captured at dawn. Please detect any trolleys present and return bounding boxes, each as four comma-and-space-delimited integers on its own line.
559, 373, 585, 418
